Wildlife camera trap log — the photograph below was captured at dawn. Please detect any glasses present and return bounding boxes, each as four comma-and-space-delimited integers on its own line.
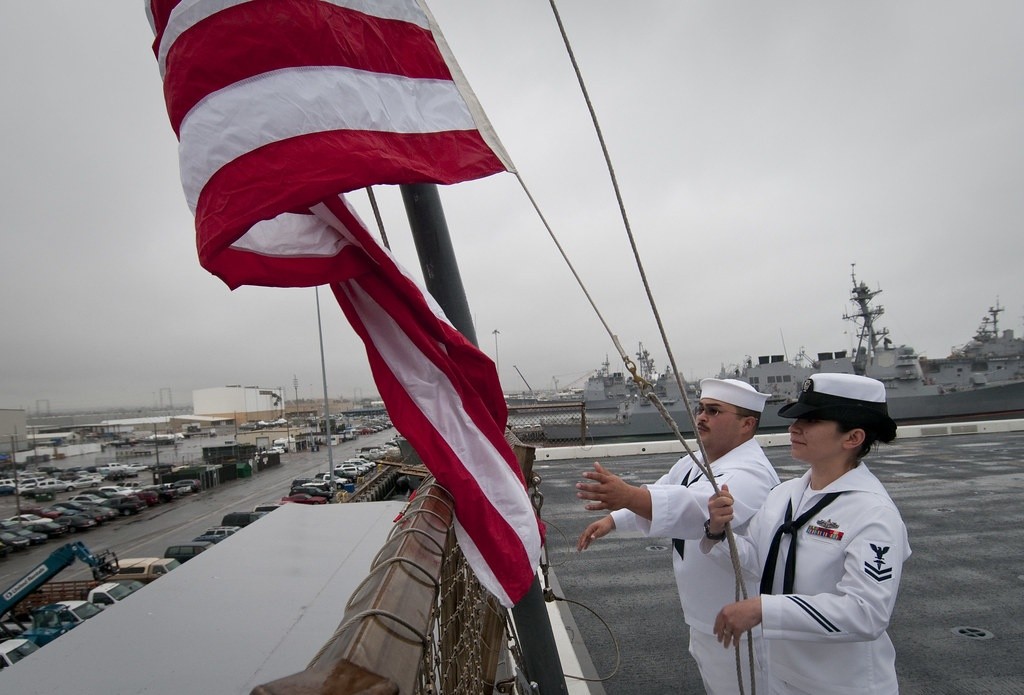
695, 401, 759, 421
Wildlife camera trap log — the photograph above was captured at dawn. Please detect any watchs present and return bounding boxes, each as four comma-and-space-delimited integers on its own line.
704, 519, 727, 542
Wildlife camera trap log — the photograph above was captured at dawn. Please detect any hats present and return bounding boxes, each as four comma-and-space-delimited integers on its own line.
777, 372, 897, 432
700, 377, 773, 413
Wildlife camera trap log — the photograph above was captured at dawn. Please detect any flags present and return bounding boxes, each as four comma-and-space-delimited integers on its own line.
144, 0, 546, 609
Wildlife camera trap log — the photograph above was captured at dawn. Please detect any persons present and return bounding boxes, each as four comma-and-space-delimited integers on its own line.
575, 380, 775, 695
700, 372, 912, 695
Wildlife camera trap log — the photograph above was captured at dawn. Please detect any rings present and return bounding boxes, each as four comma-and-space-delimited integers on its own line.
724, 629, 732, 637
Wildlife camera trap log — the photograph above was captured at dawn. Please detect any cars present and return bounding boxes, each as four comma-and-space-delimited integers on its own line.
0, 415, 407, 669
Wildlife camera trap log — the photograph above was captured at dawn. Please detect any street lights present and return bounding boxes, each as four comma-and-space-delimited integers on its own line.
492, 329, 501, 374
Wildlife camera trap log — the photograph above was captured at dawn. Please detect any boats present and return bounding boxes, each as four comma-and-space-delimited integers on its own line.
506, 263, 1024, 442
